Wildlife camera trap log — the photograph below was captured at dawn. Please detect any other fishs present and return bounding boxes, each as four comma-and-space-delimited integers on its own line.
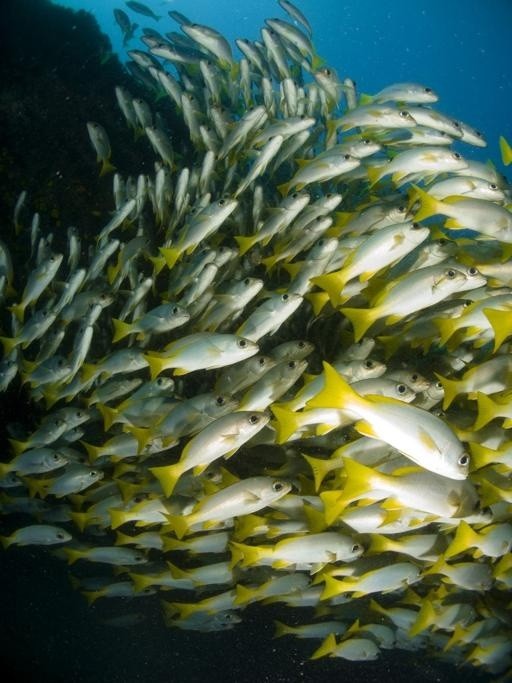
0, 0, 511, 683
